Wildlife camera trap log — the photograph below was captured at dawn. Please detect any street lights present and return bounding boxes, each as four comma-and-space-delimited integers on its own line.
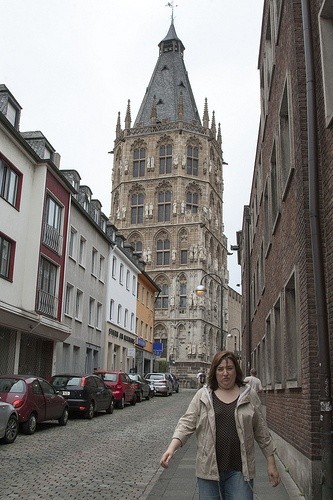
194, 272, 224, 351
227, 327, 240, 366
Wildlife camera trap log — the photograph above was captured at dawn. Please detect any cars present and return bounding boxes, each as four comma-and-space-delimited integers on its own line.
48, 373, 114, 419
0, 374, 69, 435
126, 372, 151, 401
145, 372, 180, 397
0, 402, 20, 444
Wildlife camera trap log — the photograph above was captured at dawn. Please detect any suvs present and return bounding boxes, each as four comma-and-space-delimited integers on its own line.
92, 369, 142, 410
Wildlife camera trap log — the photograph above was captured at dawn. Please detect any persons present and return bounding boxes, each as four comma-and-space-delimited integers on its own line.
160, 351, 280, 500
196, 370, 205, 389
243, 367, 264, 394
